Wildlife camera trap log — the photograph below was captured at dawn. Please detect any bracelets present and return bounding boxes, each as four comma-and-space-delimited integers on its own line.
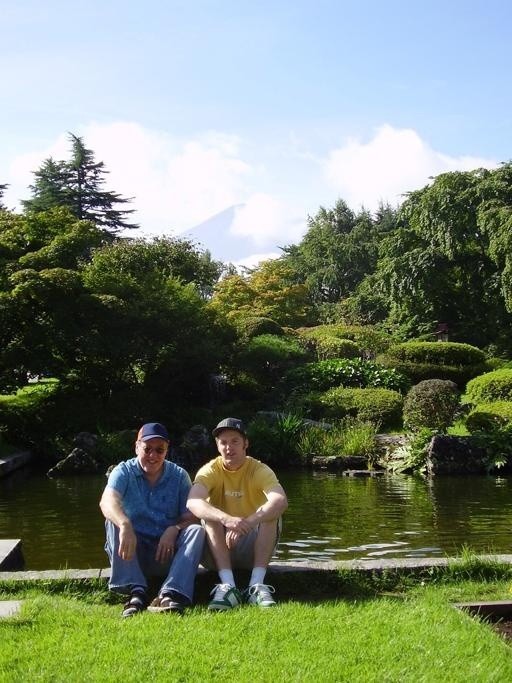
175, 525, 181, 530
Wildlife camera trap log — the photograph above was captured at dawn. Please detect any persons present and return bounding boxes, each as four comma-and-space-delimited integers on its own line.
99, 423, 205, 617
185, 418, 288, 610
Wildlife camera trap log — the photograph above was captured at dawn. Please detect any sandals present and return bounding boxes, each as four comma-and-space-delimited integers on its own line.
121, 592, 146, 617
147, 594, 183, 616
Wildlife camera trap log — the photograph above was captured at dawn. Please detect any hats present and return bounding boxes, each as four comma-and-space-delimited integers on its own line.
137, 423, 169, 444
212, 418, 248, 437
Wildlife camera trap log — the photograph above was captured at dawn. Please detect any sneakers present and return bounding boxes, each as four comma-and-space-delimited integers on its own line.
207, 584, 244, 612
243, 584, 277, 609
